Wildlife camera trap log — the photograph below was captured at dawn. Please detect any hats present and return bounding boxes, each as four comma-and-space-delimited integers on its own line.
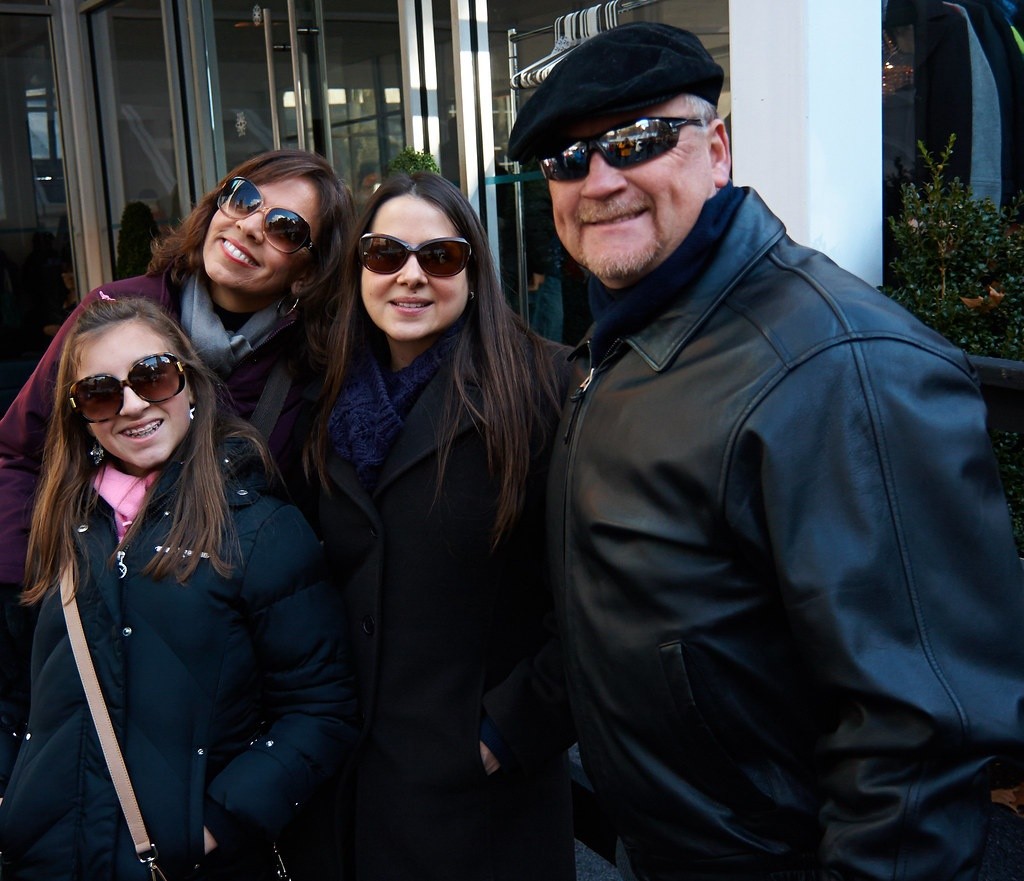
507, 22, 724, 165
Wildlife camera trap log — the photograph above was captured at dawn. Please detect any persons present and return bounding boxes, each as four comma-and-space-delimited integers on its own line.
0, 214, 78, 352
0, 149, 357, 880
507, 24, 1024, 880
0, 296, 353, 880
116, 202, 161, 280
287, 173, 581, 881
528, 188, 568, 342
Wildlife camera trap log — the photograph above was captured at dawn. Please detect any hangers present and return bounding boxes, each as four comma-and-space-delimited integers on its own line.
509, 0, 625, 91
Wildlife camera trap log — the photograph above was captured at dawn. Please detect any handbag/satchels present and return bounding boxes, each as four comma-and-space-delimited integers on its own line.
151, 838, 296, 881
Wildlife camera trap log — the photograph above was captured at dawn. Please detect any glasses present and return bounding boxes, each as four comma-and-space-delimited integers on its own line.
217, 176, 319, 262
358, 233, 472, 277
69, 351, 186, 422
539, 116, 703, 181
